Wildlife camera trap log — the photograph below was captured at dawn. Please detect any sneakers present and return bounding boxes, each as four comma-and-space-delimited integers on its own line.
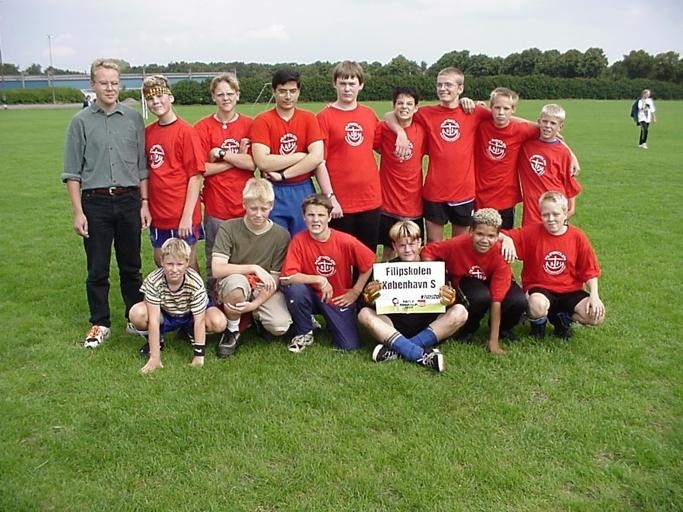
125, 321, 148, 336
458, 322, 479, 344
83, 325, 110, 349
372, 344, 403, 362
641, 143, 648, 150
310, 314, 321, 329
498, 326, 519, 342
286, 330, 313, 352
254, 321, 276, 344
176, 329, 206, 348
417, 349, 443, 372
139, 338, 165, 354
547, 309, 573, 337
531, 322, 546, 340
215, 328, 240, 358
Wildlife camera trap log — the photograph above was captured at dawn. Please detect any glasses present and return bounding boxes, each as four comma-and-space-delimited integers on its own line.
94, 81, 120, 86
435, 83, 461, 87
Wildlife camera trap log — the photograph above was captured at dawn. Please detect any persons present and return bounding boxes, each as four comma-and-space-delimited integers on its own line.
210, 177, 292, 360
636, 87, 658, 150
250, 69, 326, 239
276, 192, 378, 354
383, 67, 565, 244
315, 60, 384, 317
83, 94, 91, 109
358, 220, 468, 373
458, 87, 580, 230
419, 207, 528, 353
129, 237, 227, 375
60, 58, 153, 350
475, 99, 581, 229
192, 73, 255, 293
142, 72, 207, 278
372, 86, 428, 263
498, 191, 606, 342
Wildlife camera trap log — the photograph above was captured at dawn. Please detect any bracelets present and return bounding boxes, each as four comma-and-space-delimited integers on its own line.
192, 343, 206, 356
279, 171, 286, 181
140, 197, 148, 202
318, 277, 330, 292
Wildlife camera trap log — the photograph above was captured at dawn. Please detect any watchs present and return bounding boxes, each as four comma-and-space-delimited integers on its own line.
218, 148, 226, 159
327, 191, 335, 199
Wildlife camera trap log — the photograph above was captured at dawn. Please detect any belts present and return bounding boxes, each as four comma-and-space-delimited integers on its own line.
91, 187, 137, 195
